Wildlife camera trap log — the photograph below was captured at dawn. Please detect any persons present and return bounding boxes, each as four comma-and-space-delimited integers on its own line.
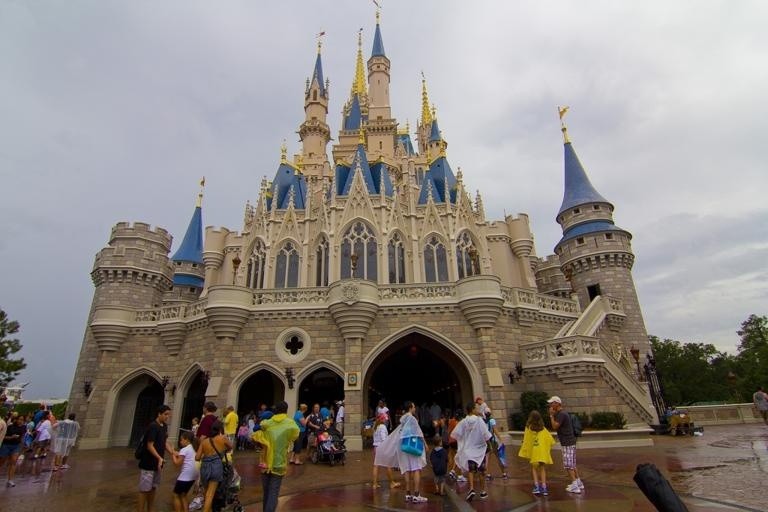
136, 405, 178, 512
372, 413, 401, 489
449, 402, 493, 501
484, 408, 508, 480
223, 404, 240, 456
415, 403, 458, 452
518, 411, 556, 496
546, 396, 584, 494
191, 418, 199, 438
335, 400, 345, 438
237, 420, 249, 451
753, 386, 768, 426
450, 462, 469, 482
171, 431, 198, 512
374, 401, 430, 503
430, 436, 450, 496
253, 411, 273, 468
245, 410, 257, 424
323, 431, 338, 451
0, 403, 82, 488
255, 404, 268, 453
476, 397, 488, 416
250, 399, 300, 512
376, 399, 393, 431
305, 404, 323, 461
288, 403, 310, 465
195, 424, 233, 512
320, 401, 331, 429
663, 405, 689, 439
196, 401, 224, 439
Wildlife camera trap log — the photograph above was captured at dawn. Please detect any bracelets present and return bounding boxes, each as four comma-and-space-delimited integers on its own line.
549, 415, 554, 418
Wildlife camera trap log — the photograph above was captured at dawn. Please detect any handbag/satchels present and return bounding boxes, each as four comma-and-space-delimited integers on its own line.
498, 443, 505, 466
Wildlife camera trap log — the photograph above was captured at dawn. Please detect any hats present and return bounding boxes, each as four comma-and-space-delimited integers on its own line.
336, 401, 342, 405
547, 396, 561, 403
485, 407, 491, 414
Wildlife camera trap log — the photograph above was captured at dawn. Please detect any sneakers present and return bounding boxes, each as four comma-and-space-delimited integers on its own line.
61, 465, 70, 469
434, 489, 439, 493
532, 486, 540, 494
373, 484, 381, 490
390, 482, 401, 489
406, 495, 414, 501
503, 473, 509, 480
53, 465, 60, 471
440, 492, 446, 495
6, 480, 16, 488
566, 479, 584, 493
413, 494, 428, 503
541, 487, 548, 496
448, 469, 493, 501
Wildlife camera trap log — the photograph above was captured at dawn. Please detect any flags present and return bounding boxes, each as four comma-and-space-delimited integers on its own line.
200, 176, 205, 187
558, 106, 569, 119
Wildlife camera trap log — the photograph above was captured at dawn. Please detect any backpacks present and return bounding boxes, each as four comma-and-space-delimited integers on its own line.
134, 431, 147, 460
571, 415, 583, 437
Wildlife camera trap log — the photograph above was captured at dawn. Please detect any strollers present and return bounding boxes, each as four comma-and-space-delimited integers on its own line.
312, 429, 347, 465
214, 449, 245, 511
